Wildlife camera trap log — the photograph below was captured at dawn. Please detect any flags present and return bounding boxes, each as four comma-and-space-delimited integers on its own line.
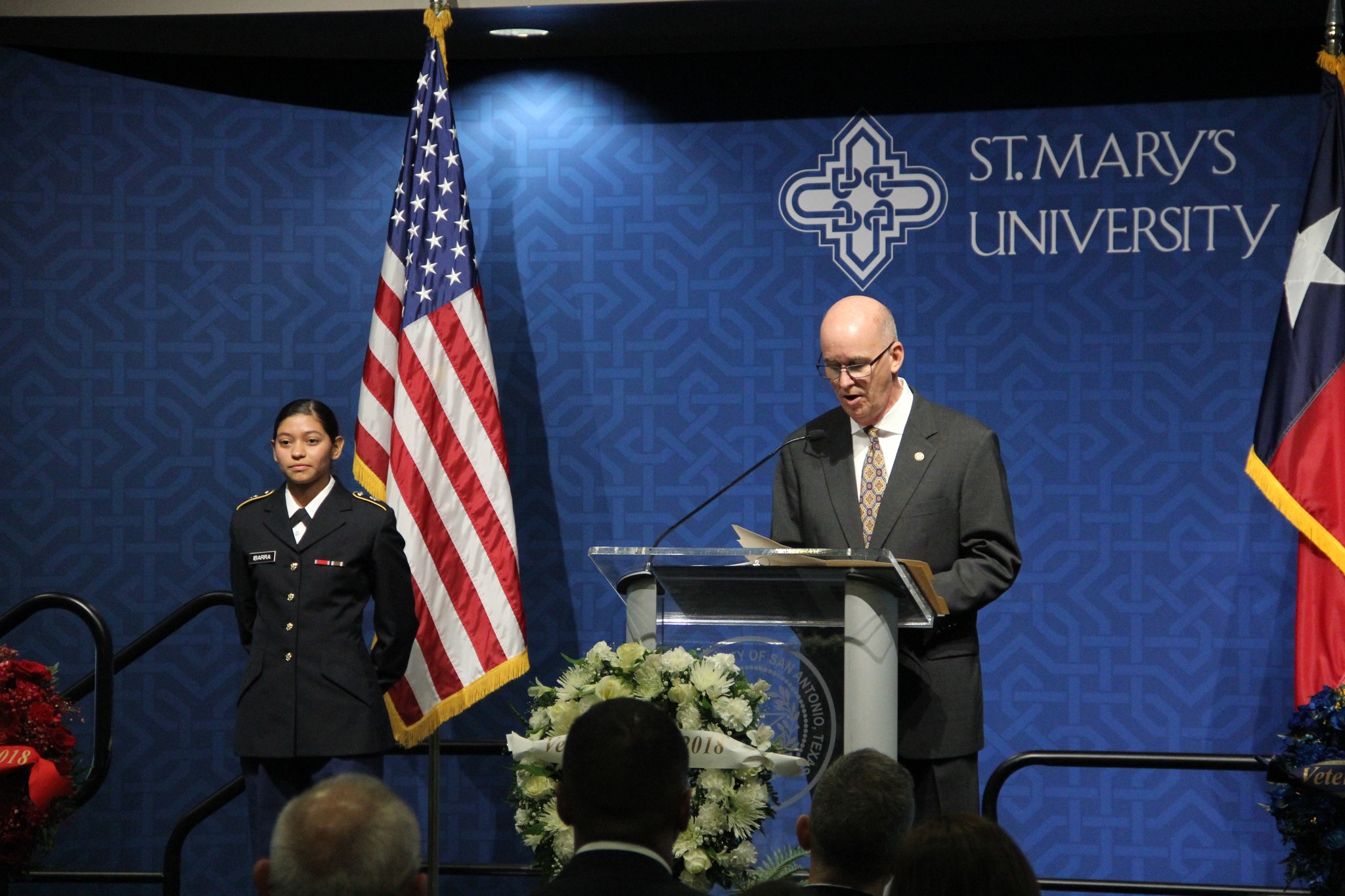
344, 22, 535, 755
1244, 203, 1345, 766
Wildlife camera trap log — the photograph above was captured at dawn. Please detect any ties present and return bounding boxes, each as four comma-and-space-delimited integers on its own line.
859, 427, 888, 549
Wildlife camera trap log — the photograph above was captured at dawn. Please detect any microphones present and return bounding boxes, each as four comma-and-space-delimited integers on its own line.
641, 428, 847, 556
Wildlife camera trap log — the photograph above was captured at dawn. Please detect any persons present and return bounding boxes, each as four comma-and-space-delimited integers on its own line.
233, 762, 433, 896
881, 812, 1045, 896
227, 398, 422, 853
758, 295, 1023, 835
735, 743, 922, 896
510, 697, 717, 896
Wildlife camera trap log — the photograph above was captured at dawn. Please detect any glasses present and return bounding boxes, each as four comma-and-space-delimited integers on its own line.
817, 342, 894, 378
